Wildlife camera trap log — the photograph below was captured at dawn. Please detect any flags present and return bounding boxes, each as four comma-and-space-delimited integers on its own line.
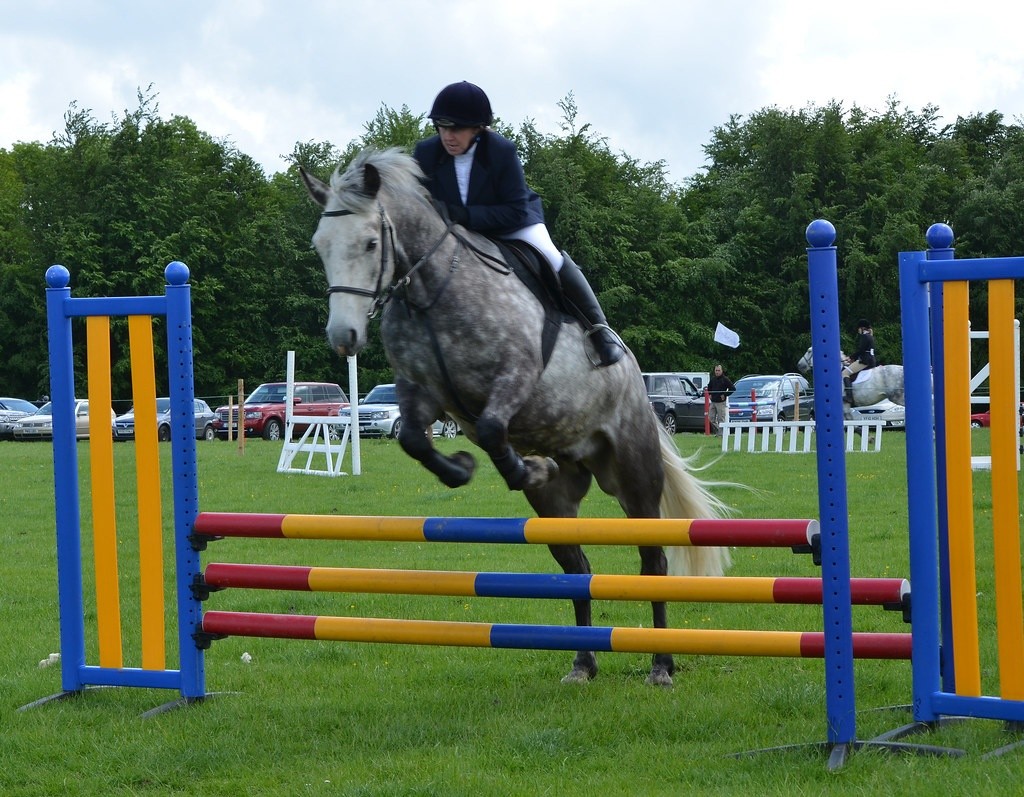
714, 322, 741, 349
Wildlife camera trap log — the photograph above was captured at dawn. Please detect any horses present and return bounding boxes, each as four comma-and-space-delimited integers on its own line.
796, 346, 936, 445
298, 144, 763, 685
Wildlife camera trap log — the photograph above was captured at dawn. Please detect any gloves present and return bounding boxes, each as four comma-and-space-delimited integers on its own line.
450, 209, 467, 228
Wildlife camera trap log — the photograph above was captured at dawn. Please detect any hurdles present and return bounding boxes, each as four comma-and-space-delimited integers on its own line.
273, 346, 363, 477
188, 498, 915, 664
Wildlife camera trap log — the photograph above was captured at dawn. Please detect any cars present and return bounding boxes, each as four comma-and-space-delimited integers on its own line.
12, 398, 117, 442
971, 408, 1024, 430
852, 399, 905, 432
642, 373, 713, 436
338, 383, 463, 441
115, 398, 216, 443
0, 397, 40, 436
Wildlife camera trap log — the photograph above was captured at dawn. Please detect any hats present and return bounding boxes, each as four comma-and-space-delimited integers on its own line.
857, 320, 870, 328
428, 81, 493, 126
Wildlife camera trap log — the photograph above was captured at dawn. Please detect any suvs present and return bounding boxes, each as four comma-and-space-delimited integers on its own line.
729, 373, 814, 435
213, 382, 351, 442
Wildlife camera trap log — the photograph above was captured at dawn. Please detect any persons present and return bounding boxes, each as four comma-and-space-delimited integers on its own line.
842, 319, 876, 404
707, 363, 736, 437
413, 79, 625, 367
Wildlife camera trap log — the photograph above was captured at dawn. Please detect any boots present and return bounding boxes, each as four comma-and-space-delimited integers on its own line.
558, 251, 626, 366
842, 377, 853, 402
